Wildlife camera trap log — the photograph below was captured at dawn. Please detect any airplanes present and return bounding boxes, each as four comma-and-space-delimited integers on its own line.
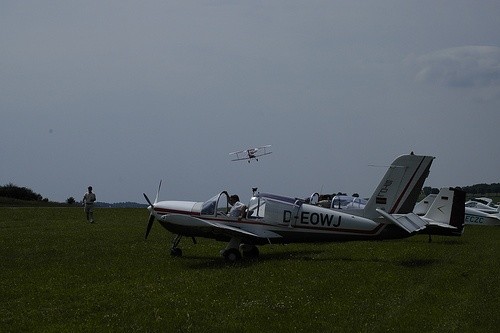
229, 143, 274, 164
142, 150, 436, 267
302, 185, 466, 244
464, 196, 500, 227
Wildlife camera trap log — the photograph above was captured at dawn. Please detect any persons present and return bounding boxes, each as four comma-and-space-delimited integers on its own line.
224, 194, 247, 219
82, 186, 97, 222
318, 195, 331, 208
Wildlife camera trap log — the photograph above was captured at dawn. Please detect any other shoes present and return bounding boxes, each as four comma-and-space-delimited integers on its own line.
89, 218, 94, 222
